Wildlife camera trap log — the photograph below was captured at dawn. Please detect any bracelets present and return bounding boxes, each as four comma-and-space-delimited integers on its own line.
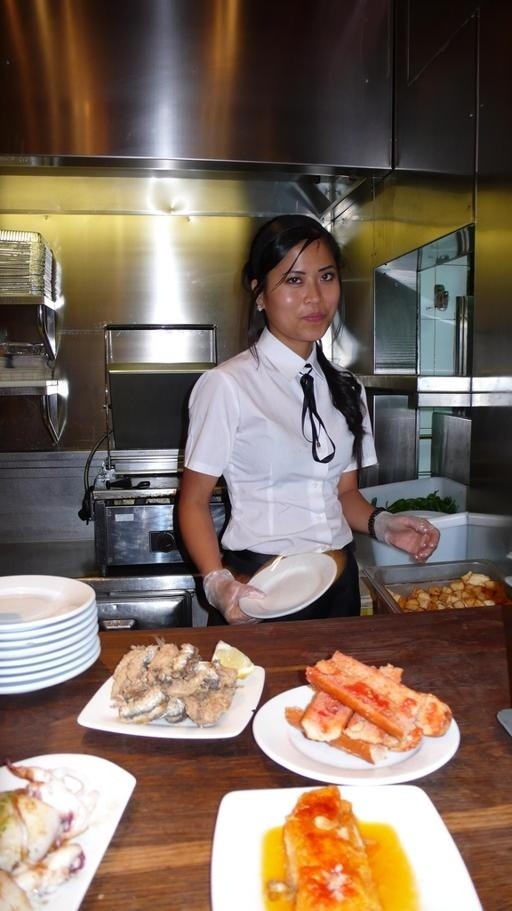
368, 507, 385, 542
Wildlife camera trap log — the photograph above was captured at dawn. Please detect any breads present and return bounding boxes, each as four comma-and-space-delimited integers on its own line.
300, 650, 453, 752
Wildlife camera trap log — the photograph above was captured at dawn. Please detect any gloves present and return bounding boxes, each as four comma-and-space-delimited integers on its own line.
202, 568, 267, 626
373, 511, 441, 565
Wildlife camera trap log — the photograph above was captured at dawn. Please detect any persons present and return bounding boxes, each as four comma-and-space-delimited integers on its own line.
171, 213, 444, 632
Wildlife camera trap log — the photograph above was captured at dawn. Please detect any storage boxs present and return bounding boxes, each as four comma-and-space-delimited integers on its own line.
352, 478, 472, 567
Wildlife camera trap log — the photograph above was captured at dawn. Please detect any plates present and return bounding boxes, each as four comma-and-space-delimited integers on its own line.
1, 573, 101, 695
77, 665, 266, 740
211, 783, 484, 911
238, 553, 338, 620
253, 685, 463, 786
1, 752, 136, 909
